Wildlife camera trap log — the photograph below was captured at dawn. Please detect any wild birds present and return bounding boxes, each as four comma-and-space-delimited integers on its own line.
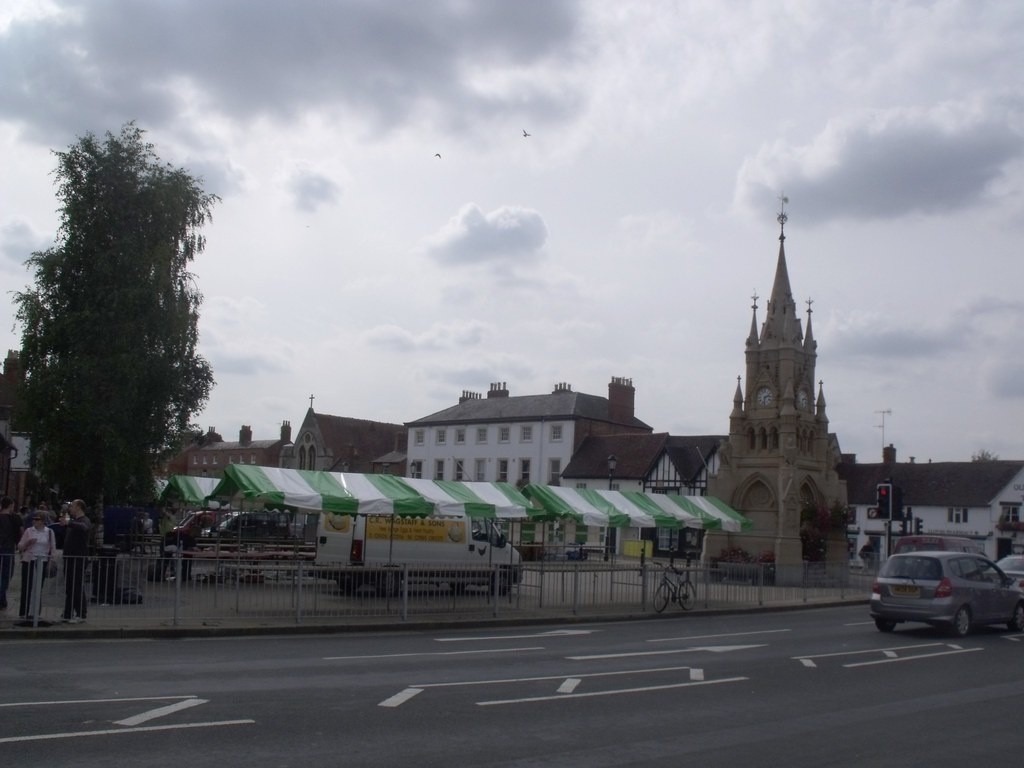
432, 152, 441, 159
522, 131, 530, 139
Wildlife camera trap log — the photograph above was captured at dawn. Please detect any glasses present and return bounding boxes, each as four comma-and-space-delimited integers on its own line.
32, 518, 42, 521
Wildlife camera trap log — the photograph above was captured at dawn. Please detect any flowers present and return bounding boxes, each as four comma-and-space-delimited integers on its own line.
718, 548, 774, 563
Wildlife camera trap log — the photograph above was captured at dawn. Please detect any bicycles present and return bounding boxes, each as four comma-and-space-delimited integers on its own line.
653, 562, 696, 612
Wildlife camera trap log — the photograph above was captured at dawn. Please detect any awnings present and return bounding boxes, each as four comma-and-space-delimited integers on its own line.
159, 475, 221, 535
204, 464, 548, 559
520, 484, 754, 559
154, 479, 169, 500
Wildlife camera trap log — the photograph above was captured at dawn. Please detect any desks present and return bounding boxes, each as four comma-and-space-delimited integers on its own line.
510, 544, 615, 562
151, 538, 316, 587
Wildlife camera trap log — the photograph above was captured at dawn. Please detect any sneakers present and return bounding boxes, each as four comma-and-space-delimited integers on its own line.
69, 618, 87, 624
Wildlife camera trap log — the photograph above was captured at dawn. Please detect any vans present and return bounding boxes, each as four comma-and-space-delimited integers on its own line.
165, 510, 228, 550
199, 510, 292, 543
308, 510, 527, 598
894, 535, 979, 574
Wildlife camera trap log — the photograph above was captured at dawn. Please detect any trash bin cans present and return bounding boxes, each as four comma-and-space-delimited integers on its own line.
92, 546, 121, 606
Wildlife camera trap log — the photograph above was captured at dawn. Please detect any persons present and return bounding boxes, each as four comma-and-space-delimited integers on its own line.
17, 511, 56, 622
59, 499, 92, 623
8, 500, 157, 555
846, 538, 874, 576
0, 498, 18, 609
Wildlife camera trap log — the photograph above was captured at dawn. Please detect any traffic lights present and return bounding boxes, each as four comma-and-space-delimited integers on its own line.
899, 519, 905, 535
917, 518, 924, 535
906, 506, 912, 520
877, 484, 891, 521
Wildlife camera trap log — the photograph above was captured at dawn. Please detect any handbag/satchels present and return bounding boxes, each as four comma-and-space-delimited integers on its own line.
46, 560, 58, 578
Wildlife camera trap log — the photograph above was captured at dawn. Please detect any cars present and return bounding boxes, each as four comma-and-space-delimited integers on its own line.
868, 550, 1024, 640
985, 554, 1024, 595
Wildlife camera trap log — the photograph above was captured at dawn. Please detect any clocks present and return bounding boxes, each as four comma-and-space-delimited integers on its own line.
798, 390, 809, 409
756, 387, 773, 407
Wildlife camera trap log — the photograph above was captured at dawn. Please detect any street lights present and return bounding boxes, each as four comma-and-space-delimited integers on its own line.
410, 459, 416, 479
602, 454, 617, 562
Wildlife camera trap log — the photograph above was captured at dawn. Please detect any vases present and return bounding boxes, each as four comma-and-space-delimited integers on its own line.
718, 562, 774, 587
